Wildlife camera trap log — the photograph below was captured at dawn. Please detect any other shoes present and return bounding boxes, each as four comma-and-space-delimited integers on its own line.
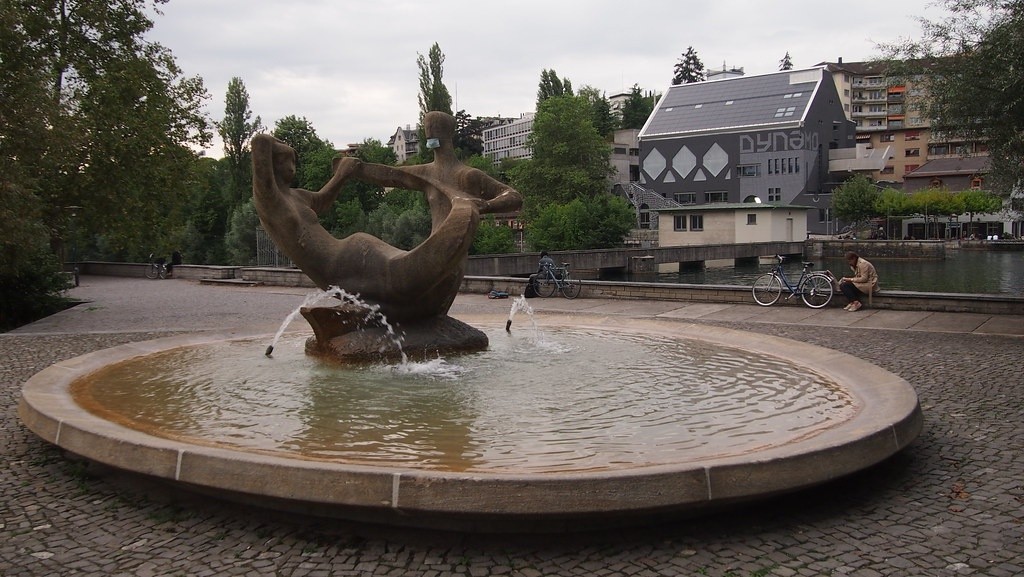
166, 273, 172, 276
844, 302, 862, 311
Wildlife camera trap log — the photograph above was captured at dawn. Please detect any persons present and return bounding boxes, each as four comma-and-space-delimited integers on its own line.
840, 252, 880, 311
529, 250, 556, 278
251, 111, 522, 336
163, 243, 182, 275
970, 232, 982, 240
50, 229, 64, 271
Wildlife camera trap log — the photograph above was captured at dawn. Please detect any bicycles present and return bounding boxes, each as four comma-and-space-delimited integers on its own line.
751, 252, 835, 309
144, 253, 167, 280
533, 263, 582, 300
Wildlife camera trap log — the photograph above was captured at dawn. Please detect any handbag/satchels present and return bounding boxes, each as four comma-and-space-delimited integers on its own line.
525, 282, 540, 297
154, 256, 167, 264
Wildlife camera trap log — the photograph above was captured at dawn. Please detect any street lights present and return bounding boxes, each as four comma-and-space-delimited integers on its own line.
64, 206, 83, 287
